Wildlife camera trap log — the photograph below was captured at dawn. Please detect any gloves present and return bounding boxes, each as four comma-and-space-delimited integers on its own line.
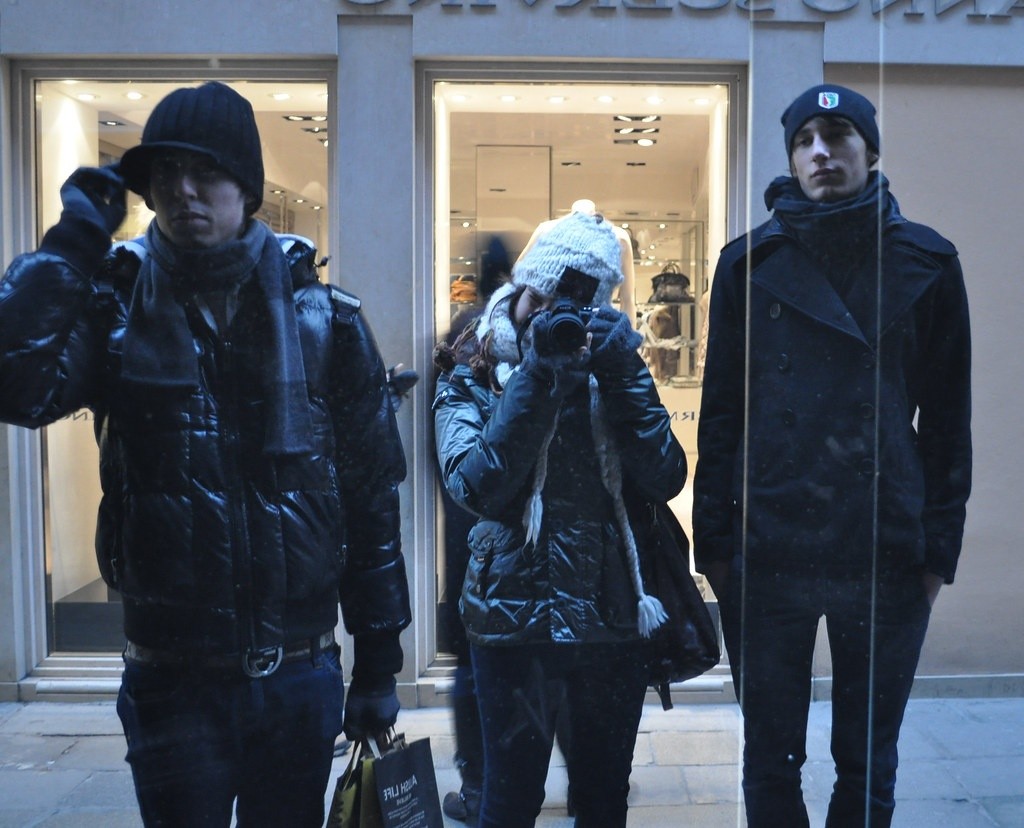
60, 164, 128, 234
343, 678, 400, 743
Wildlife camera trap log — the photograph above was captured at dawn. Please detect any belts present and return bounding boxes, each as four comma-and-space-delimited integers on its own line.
117, 629, 340, 679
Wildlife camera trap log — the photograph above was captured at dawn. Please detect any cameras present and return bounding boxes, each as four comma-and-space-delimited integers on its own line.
533, 297, 601, 356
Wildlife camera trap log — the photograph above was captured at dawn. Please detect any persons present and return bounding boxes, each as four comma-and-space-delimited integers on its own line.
436, 229, 585, 824
681, 79, 974, 828
429, 202, 690, 828
0, 74, 413, 828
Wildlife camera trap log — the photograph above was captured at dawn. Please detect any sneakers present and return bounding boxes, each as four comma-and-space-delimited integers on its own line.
444, 791, 483, 827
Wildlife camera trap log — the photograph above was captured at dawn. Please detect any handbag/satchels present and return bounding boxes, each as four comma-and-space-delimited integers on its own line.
632, 491, 722, 712
368, 725, 443, 828
360, 733, 408, 828
328, 741, 360, 828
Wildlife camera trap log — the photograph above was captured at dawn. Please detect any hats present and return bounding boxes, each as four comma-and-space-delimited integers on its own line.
511, 209, 624, 310
119, 80, 264, 224
781, 84, 880, 180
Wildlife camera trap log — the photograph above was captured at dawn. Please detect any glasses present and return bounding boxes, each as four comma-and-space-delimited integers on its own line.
142, 158, 228, 190
